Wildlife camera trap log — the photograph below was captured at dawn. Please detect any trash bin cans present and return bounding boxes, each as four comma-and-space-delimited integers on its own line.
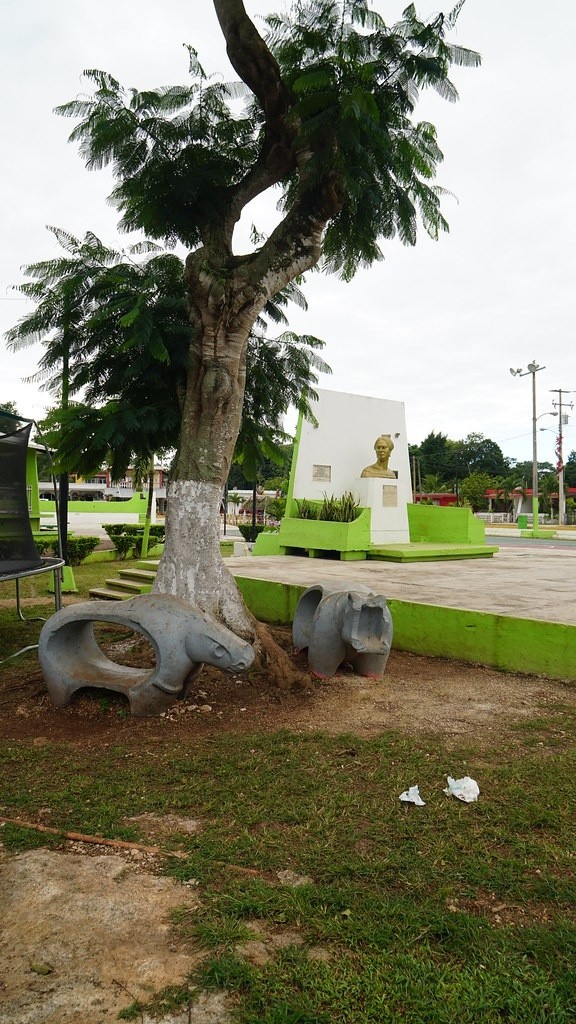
517, 514, 528, 528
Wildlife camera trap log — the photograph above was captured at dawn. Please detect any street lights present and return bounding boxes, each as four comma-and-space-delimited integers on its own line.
540, 388, 576, 526
509, 359, 558, 529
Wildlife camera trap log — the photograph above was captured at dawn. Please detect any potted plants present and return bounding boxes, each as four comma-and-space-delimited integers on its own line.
233, 524, 265, 556
406, 496, 486, 544
278, 490, 372, 560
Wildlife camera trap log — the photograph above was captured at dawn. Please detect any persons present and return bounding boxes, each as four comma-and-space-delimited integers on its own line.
361, 435, 397, 479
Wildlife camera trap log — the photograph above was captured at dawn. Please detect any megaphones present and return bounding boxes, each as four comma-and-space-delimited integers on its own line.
510, 368, 522, 376
528, 363, 539, 372
